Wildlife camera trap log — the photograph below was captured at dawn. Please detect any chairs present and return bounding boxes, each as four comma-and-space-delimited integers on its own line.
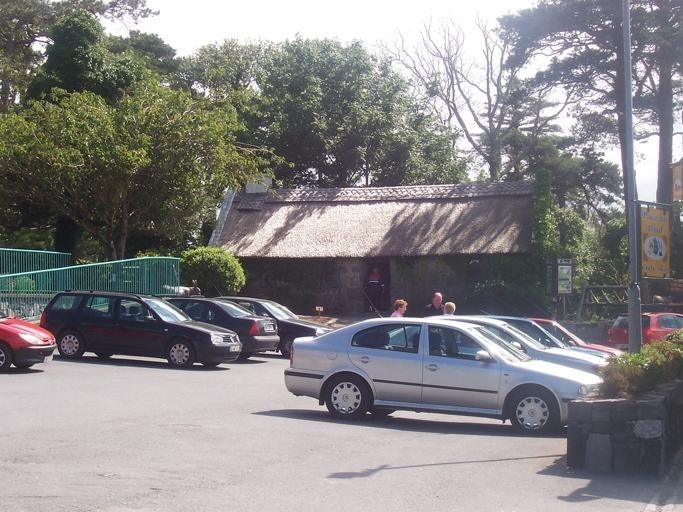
376, 330, 395, 351
429, 332, 444, 356
447, 335, 461, 358
412, 333, 420, 354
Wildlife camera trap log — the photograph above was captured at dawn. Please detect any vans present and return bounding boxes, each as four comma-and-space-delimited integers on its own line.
39, 289, 243, 370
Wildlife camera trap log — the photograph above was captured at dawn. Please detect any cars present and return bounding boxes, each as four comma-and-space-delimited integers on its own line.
0, 309, 57, 372
387, 315, 609, 379
213, 297, 336, 359
502, 317, 626, 364
283, 319, 605, 436
160, 298, 280, 363
607, 313, 683, 351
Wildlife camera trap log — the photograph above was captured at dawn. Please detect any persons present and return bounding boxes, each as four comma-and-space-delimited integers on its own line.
421, 290, 445, 317
442, 301, 456, 315
364, 265, 387, 312
389, 298, 408, 318
